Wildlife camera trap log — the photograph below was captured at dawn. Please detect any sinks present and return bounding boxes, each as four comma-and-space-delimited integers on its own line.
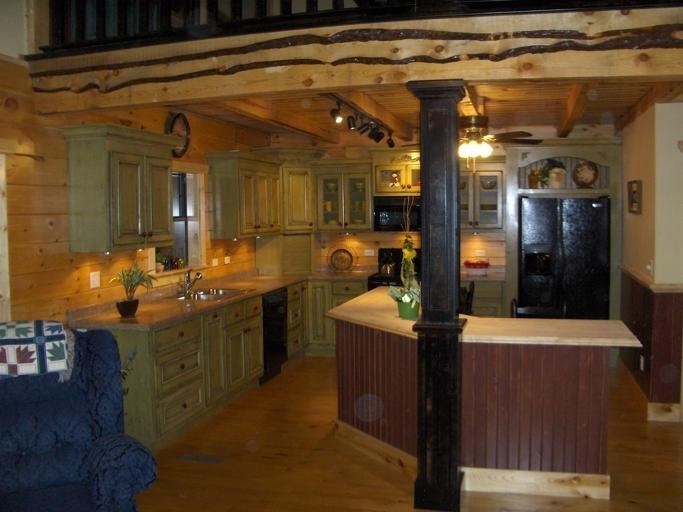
203, 288, 241, 295
179, 294, 225, 302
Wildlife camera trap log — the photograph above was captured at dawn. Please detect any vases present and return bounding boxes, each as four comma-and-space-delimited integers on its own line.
398, 300, 419, 320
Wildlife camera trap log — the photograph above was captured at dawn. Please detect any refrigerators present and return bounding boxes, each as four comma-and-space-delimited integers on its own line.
518, 194, 611, 319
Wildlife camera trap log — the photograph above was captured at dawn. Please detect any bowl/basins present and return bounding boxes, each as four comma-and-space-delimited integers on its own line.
327, 183, 337, 192
354, 182, 365, 191
480, 179, 496, 190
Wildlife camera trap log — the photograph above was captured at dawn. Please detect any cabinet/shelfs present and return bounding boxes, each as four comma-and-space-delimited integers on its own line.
204, 309, 223, 402
207, 149, 281, 240
455, 170, 504, 231
155, 316, 206, 437
279, 157, 315, 234
305, 279, 366, 351
62, 122, 175, 253
264, 282, 305, 376
312, 158, 374, 231
222, 294, 264, 391
505, 139, 624, 318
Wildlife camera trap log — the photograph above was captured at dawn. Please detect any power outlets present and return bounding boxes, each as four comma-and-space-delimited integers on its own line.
212, 258, 219, 267
89, 270, 102, 289
475, 249, 487, 257
363, 248, 375, 258
224, 256, 232, 265
638, 354, 645, 371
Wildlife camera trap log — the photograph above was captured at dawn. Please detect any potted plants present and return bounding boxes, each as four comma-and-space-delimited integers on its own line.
108, 261, 157, 318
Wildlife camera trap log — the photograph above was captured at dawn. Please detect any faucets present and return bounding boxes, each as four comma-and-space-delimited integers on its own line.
184, 268, 202, 296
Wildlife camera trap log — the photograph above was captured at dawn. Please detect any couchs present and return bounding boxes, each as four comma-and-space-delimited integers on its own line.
0, 319, 163, 512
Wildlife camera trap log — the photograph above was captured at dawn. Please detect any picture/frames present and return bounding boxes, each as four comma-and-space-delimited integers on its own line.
376, 165, 406, 192
407, 164, 421, 191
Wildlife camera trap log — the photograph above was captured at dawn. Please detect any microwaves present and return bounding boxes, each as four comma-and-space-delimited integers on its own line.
373, 196, 421, 230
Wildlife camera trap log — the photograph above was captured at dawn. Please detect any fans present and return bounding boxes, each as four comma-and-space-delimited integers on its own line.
458, 115, 544, 146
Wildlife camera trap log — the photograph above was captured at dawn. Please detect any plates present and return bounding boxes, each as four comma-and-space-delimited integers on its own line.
352, 219, 364, 224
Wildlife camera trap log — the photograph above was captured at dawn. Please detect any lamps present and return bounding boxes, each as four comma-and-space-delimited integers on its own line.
368, 124, 379, 139
347, 112, 358, 131
330, 100, 344, 124
373, 130, 384, 142
457, 117, 497, 174
358, 120, 374, 134
387, 129, 395, 148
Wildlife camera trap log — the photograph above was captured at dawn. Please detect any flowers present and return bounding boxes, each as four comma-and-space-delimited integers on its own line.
388, 184, 420, 303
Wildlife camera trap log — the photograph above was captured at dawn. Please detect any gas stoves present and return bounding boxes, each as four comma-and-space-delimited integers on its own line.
368, 272, 421, 292
